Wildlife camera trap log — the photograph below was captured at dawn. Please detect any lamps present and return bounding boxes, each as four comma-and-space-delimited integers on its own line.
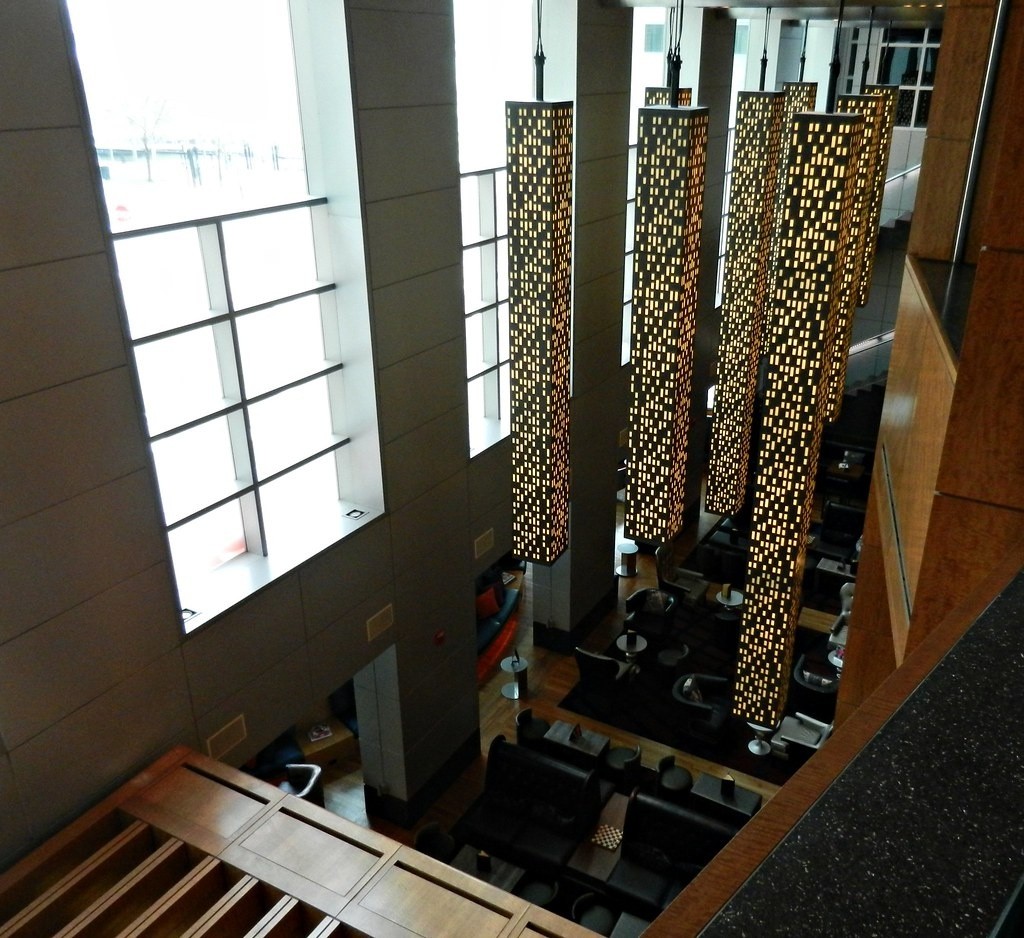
499, 81, 899, 742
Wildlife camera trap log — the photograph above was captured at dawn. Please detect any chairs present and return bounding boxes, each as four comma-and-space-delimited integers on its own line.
414, 536, 838, 936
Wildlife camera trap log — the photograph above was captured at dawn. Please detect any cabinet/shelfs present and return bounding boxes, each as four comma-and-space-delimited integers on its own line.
1, 746, 609, 938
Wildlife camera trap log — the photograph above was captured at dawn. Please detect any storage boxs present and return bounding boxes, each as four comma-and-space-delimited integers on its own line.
296, 716, 359, 779
